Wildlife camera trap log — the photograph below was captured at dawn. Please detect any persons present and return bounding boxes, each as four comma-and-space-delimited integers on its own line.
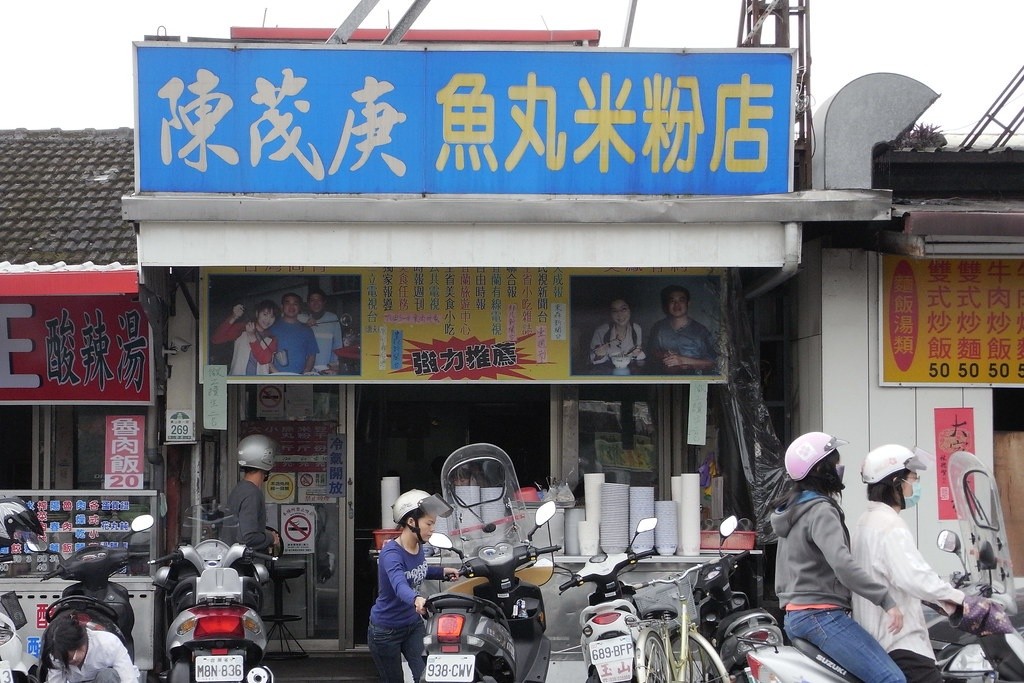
771, 432, 907, 683
41, 611, 140, 683
367, 489, 458, 683
588, 287, 716, 370
455, 467, 487, 488
852, 444, 965, 683
220, 435, 279, 613
212, 289, 342, 375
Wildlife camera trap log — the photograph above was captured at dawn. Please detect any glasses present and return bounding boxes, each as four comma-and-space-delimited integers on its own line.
908, 475, 920, 483
53, 650, 79, 665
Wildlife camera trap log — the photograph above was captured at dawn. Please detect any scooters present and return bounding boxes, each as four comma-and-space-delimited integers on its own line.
693, 450, 1023, 683
556, 517, 660, 683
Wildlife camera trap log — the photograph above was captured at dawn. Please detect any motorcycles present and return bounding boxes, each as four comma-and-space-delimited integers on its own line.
419, 443, 562, 683
0, 538, 46, 683
147, 505, 275, 683
25, 515, 154, 665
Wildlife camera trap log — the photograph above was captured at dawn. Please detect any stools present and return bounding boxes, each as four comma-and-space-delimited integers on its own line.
261, 526, 311, 661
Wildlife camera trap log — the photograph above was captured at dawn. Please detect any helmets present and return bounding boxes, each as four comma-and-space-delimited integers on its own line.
784, 433, 848, 481
861, 444, 933, 488
236, 435, 278, 472
0, 497, 44, 546
392, 489, 453, 525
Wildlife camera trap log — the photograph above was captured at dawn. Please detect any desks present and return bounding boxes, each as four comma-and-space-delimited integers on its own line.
376, 554, 721, 661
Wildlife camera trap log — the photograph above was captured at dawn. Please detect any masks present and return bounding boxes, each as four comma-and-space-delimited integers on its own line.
897, 478, 921, 508
832, 464, 845, 480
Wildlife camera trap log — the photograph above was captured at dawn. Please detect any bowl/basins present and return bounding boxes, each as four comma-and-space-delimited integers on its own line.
381, 473, 701, 556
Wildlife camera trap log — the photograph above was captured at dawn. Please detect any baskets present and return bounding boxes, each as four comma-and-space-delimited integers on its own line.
632, 573, 698, 632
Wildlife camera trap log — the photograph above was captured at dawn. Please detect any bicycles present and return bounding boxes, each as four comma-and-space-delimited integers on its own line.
625, 558, 731, 683
